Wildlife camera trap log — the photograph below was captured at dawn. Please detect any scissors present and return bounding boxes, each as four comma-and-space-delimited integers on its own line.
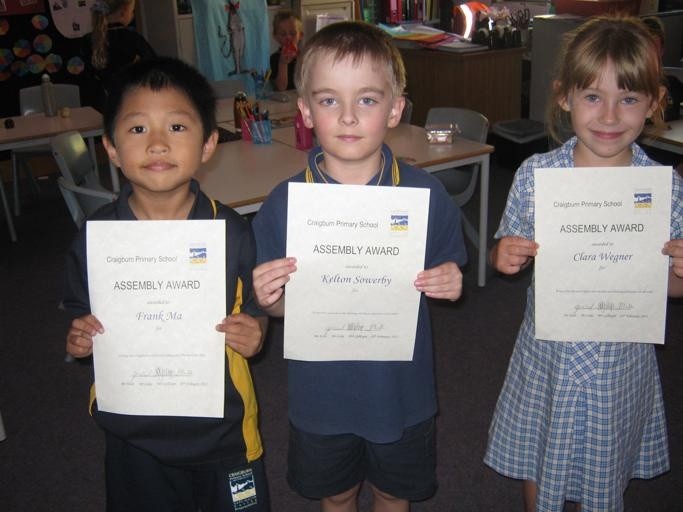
518, 7, 530, 29
505, 12, 518, 29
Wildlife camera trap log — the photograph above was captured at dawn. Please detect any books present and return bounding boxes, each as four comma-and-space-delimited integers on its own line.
389, 28, 452, 49
355, 0, 445, 25
437, 42, 488, 54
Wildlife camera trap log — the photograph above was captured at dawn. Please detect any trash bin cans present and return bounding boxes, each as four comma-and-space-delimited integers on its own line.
492, 118, 550, 191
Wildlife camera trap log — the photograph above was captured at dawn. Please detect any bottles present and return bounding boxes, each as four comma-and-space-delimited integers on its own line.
254, 76, 264, 99
41, 74, 57, 117
294, 110, 313, 151
233, 92, 247, 127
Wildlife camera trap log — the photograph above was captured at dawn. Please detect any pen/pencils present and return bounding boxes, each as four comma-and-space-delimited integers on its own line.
235, 95, 270, 144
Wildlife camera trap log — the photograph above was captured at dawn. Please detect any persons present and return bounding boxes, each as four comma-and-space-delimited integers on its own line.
249, 18, 467, 510
51, 53, 272, 512
482, 10, 682, 511
65, 0, 159, 81
266, 7, 305, 92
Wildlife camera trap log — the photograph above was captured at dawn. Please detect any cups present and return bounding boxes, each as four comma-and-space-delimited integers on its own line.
240, 119, 251, 143
249, 120, 271, 145
